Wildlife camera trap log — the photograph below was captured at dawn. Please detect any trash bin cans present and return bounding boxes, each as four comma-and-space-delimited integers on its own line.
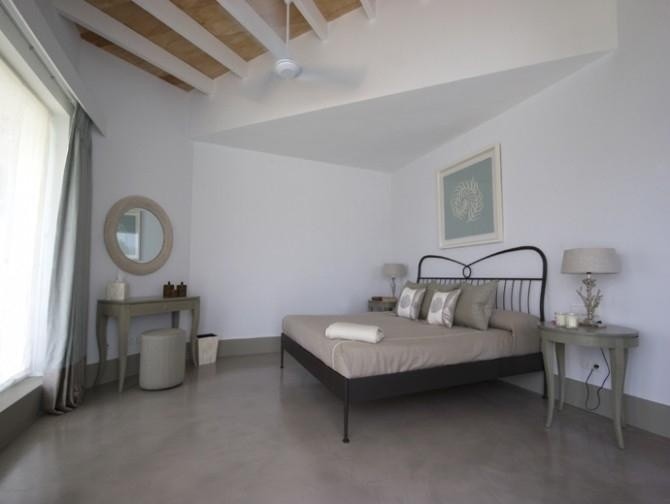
196, 332, 218, 365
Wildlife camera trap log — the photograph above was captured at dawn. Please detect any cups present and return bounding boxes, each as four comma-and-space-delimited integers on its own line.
555, 312, 580, 329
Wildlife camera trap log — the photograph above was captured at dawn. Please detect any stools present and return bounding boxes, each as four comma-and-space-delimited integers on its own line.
138, 327, 186, 389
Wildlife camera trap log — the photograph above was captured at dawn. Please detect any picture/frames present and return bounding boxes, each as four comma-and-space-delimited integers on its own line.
434, 142, 503, 250
116, 208, 141, 261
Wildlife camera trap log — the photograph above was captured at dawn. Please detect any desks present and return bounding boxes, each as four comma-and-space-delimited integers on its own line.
368, 298, 397, 312
91, 294, 200, 392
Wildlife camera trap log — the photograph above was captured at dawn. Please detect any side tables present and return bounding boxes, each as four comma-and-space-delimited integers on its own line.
537, 319, 639, 451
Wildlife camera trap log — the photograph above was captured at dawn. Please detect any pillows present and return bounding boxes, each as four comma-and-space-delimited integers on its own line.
391, 279, 500, 331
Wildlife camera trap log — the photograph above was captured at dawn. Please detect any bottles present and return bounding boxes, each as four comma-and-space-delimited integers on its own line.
163, 281, 187, 297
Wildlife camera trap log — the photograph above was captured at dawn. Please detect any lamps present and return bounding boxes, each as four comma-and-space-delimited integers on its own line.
383, 264, 408, 297
561, 250, 622, 328
275, 0, 303, 79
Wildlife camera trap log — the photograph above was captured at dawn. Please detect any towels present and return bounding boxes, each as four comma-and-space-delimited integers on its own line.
323, 321, 384, 343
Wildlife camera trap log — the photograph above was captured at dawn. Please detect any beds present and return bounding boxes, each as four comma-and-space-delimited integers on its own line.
278, 246, 549, 444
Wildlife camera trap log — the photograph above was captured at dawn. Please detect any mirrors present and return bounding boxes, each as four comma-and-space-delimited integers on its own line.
103, 195, 174, 276
